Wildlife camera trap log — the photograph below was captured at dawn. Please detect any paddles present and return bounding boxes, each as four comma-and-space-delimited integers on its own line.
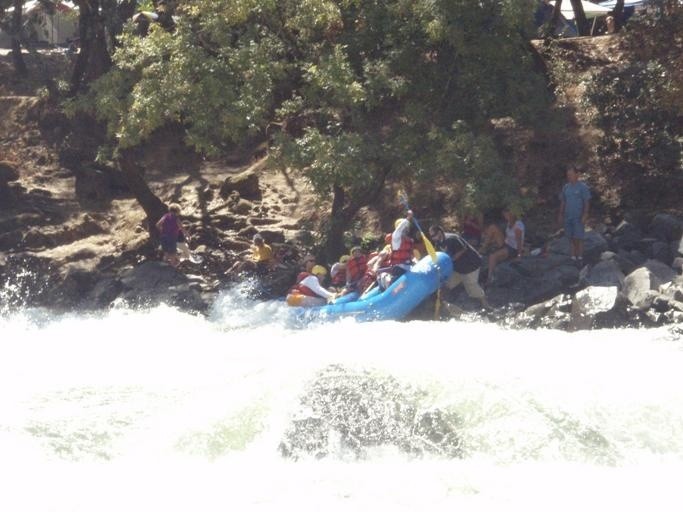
398, 189, 438, 264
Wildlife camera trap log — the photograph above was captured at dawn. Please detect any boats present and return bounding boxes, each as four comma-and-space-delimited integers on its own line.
276, 251, 453, 330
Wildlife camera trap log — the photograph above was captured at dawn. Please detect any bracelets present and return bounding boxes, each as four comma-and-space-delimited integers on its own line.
517, 254, 521, 256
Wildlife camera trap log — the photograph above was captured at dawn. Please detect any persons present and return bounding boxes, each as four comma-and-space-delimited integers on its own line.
598, 15, 622, 35
429, 226, 488, 310
559, 165, 592, 259
289, 209, 421, 305
480, 210, 526, 280
155, 203, 185, 268
223, 234, 271, 276
463, 207, 479, 241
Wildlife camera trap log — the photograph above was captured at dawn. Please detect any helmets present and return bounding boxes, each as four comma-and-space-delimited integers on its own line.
340, 246, 361, 263
169, 203, 181, 213
312, 265, 327, 276
385, 233, 392, 244
395, 218, 409, 229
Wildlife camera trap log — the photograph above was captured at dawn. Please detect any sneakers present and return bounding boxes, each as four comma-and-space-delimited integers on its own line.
480, 305, 493, 310
571, 259, 583, 267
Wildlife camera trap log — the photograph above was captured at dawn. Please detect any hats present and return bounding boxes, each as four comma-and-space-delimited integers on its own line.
301, 255, 316, 265
253, 234, 262, 241
